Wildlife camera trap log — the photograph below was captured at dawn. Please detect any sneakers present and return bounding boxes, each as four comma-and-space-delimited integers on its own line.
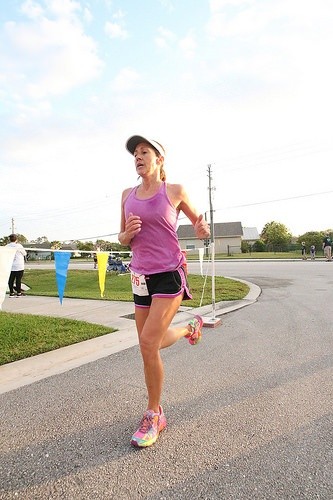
17, 293, 26, 297
185, 315, 203, 345
9, 294, 17, 297
130, 405, 167, 448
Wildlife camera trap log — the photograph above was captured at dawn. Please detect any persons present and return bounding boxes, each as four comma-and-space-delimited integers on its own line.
93, 253, 98, 268
4, 233, 28, 297
301, 241, 307, 260
310, 246, 315, 258
322, 233, 332, 258
118, 133, 211, 448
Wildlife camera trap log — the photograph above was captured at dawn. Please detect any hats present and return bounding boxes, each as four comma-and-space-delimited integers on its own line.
125, 134, 164, 157
